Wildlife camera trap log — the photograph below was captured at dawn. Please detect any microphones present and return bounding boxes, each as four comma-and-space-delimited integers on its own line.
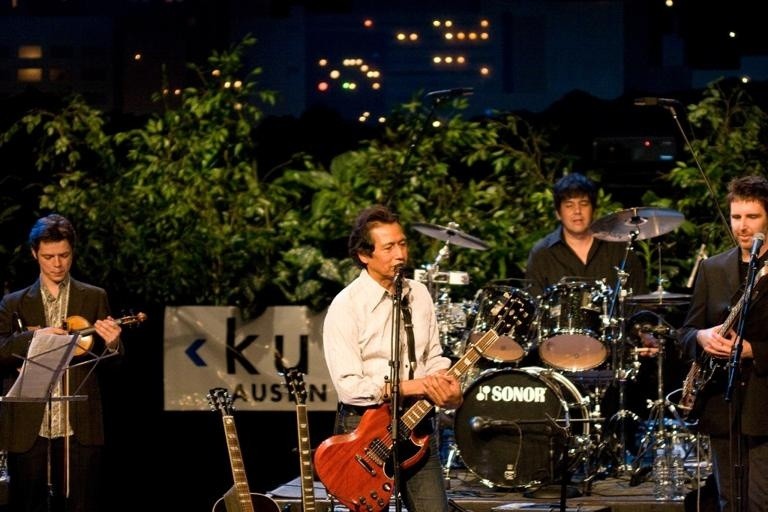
749, 232, 765, 266
392, 267, 404, 284
634, 96, 679, 107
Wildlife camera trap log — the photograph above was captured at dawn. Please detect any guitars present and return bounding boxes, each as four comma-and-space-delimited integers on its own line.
26, 313, 146, 362
283, 370, 332, 510
315, 290, 532, 511
680, 263, 767, 424
206, 386, 281, 512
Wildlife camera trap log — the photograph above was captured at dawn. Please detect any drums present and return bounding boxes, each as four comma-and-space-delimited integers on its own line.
470, 285, 536, 362
454, 366, 590, 490
537, 282, 610, 373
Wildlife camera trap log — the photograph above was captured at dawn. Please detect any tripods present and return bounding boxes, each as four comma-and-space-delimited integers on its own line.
630, 309, 710, 487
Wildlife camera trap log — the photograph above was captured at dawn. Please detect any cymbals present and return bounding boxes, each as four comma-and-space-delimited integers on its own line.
627, 293, 692, 306
412, 223, 489, 250
590, 205, 684, 242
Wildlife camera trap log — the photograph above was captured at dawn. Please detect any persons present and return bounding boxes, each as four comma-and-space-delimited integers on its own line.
321, 205, 464, 511
2, 213, 128, 511
522, 173, 646, 370
673, 173, 768, 511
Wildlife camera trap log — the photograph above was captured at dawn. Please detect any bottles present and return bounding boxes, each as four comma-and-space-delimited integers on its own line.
671, 449, 686, 501
653, 449, 669, 500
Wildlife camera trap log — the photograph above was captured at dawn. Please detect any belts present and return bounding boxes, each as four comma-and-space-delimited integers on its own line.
338, 402, 379, 415
